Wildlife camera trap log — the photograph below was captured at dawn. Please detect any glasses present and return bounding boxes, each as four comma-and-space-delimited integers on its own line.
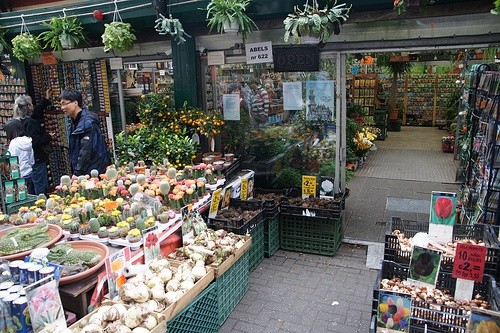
59, 101, 72, 108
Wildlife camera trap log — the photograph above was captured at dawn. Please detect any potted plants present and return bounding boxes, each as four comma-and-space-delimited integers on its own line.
0, 151, 234, 242
153, 13, 192, 44
283, 0, 352, 44
197, 0, 259, 37
41, 15, 91, 62
348, 147, 369, 171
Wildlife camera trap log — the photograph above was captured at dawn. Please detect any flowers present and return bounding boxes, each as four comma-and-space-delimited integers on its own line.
347, 116, 383, 163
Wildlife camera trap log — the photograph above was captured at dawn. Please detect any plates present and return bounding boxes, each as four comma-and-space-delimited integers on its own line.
47, 241, 109, 287
0, 223, 62, 260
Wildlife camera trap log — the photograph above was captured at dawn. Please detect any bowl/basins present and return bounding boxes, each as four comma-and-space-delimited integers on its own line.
121, 87, 143, 95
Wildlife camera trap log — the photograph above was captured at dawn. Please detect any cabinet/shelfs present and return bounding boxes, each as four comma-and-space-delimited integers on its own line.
347, 73, 461, 126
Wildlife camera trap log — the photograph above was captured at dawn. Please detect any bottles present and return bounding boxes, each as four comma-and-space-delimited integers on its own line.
133, 73, 149, 94
202, 152, 237, 168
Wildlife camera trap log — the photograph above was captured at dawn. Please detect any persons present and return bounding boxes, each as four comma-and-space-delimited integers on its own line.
60, 88, 110, 182
2, 87, 52, 198
220, 78, 269, 126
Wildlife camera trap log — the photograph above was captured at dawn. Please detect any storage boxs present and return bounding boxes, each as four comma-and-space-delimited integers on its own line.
67, 189, 344, 333
368, 216, 500, 333
442, 144, 454, 153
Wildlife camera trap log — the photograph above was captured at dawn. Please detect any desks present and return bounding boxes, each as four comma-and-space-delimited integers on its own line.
57, 169, 255, 316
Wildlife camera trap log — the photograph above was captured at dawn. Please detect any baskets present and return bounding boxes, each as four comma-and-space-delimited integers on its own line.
380, 215, 500, 333
230, 188, 282, 220
279, 188, 346, 221
206, 205, 266, 236
